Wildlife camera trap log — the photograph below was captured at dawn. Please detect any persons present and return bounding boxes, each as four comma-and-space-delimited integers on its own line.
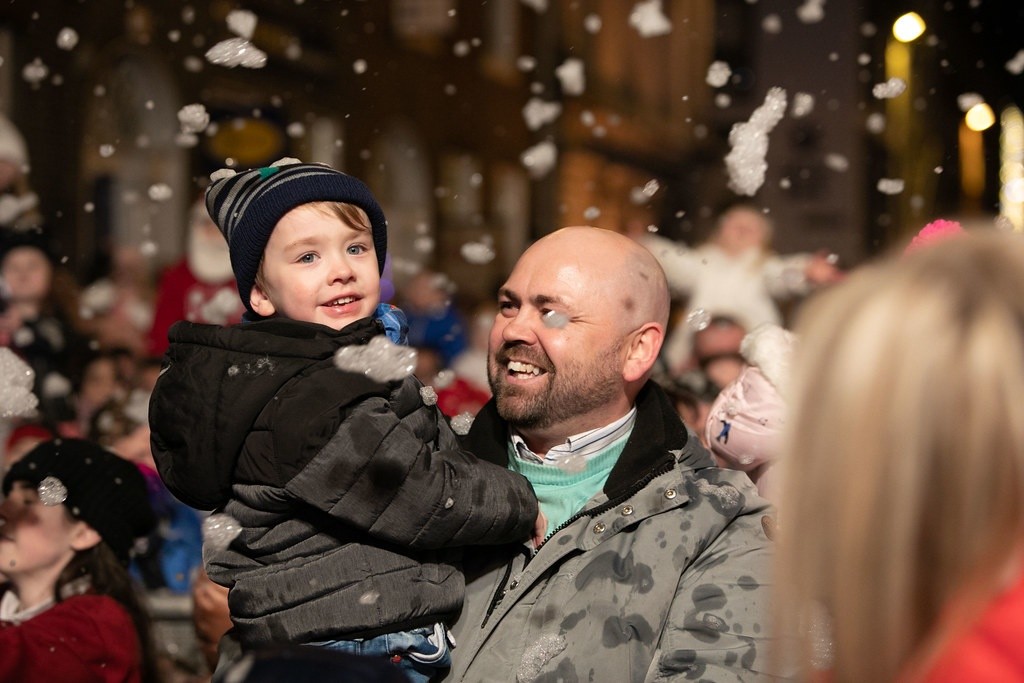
768, 227, 1024, 683
0, 107, 966, 683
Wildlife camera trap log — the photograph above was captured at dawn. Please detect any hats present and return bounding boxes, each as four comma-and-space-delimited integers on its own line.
204, 162, 391, 307
3, 439, 160, 568
